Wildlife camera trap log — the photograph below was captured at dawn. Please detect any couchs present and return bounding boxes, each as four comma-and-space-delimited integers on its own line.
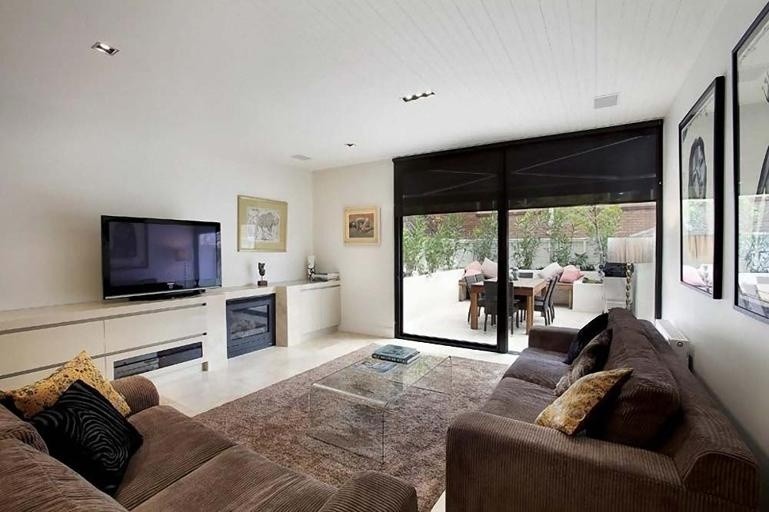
0, 375, 420, 512
458, 268, 573, 310
445, 323, 748, 512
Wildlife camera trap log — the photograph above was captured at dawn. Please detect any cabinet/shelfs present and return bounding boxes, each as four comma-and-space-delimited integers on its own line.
1, 291, 226, 387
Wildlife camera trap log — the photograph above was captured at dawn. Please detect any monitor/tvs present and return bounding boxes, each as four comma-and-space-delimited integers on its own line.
100, 214, 222, 302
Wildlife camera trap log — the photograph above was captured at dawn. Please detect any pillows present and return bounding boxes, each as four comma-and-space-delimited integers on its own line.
464, 259, 482, 274
25, 378, 145, 496
562, 312, 610, 367
536, 261, 564, 282
558, 264, 584, 284
552, 327, 615, 397
481, 256, 499, 279
533, 366, 636, 439
1, 347, 133, 422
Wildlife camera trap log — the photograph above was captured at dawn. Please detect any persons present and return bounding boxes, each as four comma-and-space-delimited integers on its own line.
688, 136, 707, 199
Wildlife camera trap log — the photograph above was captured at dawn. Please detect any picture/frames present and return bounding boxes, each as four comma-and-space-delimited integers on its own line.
677, 74, 726, 300
236, 194, 289, 255
731, 1, 768, 327
342, 204, 383, 248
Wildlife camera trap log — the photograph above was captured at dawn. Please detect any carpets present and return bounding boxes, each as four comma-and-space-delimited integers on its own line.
190, 342, 511, 512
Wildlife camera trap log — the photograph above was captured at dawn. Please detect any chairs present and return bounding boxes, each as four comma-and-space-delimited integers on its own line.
483, 279, 521, 335
464, 274, 496, 326
519, 277, 557, 326
534, 270, 564, 324
475, 274, 490, 298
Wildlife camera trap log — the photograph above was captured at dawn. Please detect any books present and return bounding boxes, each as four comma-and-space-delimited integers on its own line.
372, 344, 421, 365
